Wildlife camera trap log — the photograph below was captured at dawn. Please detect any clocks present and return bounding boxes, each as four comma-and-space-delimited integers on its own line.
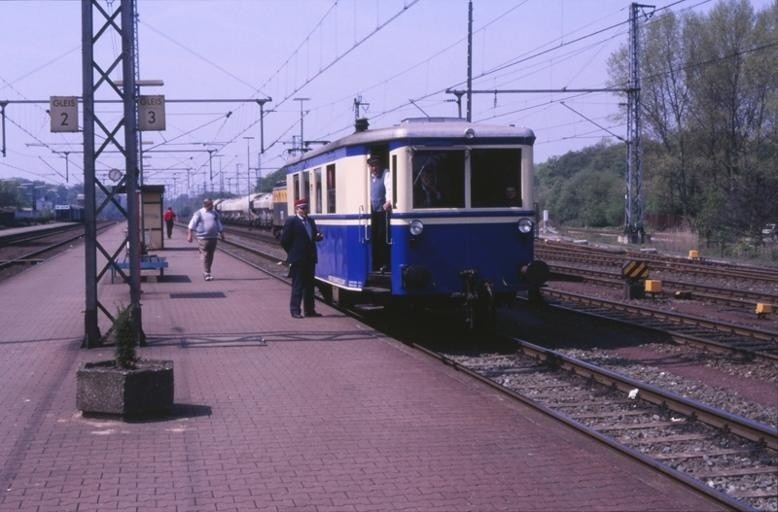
108, 169, 122, 182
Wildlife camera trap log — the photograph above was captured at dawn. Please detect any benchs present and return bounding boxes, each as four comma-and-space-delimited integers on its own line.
109, 261, 168, 284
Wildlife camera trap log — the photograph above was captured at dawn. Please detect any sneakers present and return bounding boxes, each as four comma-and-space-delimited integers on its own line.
203, 272, 214, 281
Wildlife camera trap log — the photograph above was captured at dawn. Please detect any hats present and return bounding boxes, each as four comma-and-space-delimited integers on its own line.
294, 199, 308, 208
367, 153, 384, 164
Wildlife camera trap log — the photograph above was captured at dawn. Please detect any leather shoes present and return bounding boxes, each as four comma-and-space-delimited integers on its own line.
379, 264, 391, 272
291, 311, 321, 318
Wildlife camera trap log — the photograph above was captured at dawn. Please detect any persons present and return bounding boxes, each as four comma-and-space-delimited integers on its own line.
186, 197, 226, 281
413, 162, 443, 207
366, 155, 394, 273
279, 198, 327, 320
163, 207, 176, 239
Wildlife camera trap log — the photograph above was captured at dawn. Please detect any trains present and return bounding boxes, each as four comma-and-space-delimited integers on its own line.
213, 116, 551, 331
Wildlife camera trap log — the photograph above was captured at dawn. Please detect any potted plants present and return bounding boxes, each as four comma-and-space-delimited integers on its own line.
75, 300, 174, 417
